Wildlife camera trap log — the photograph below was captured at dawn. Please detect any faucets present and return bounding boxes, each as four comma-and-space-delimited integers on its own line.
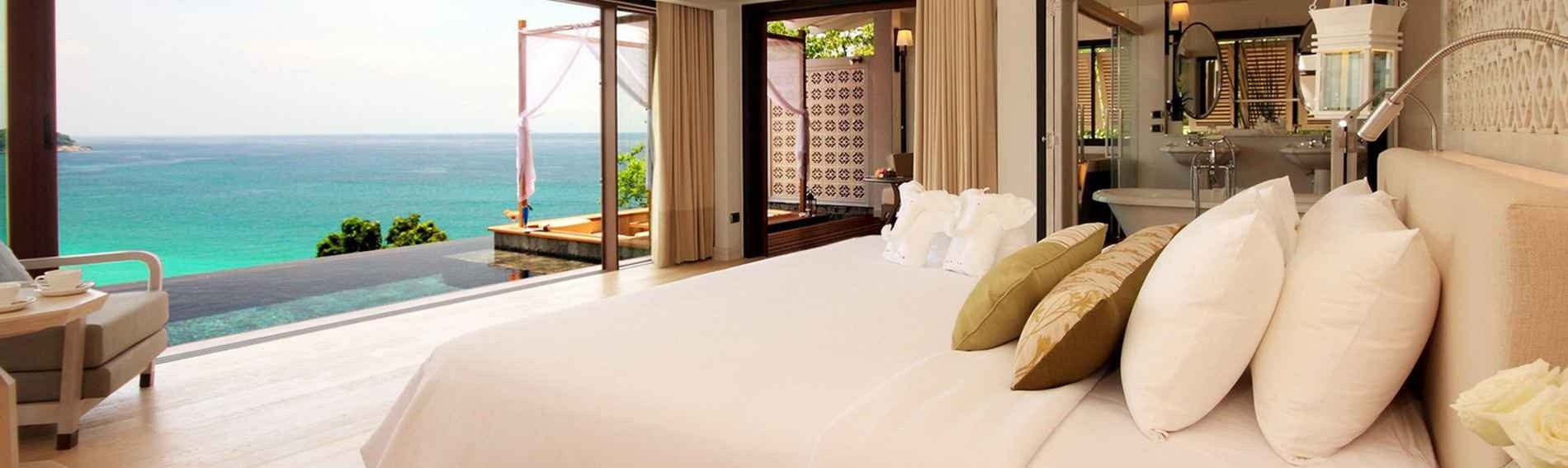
1301, 133, 1331, 149
1193, 165, 1229, 219
1186, 135, 1225, 146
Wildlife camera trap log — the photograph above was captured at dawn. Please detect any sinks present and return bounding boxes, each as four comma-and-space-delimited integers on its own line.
1280, 145, 1365, 169
1159, 144, 1241, 166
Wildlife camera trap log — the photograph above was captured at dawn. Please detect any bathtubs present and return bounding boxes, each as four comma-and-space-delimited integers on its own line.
1092, 188, 1326, 237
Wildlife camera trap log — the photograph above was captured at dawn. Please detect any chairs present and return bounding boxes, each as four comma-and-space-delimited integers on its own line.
0, 240, 169, 450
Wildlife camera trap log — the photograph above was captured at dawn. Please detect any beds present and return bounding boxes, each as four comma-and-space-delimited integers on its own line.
359, 151, 1568, 468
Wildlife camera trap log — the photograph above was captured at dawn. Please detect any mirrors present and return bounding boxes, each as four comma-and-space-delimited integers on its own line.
1173, 22, 1224, 121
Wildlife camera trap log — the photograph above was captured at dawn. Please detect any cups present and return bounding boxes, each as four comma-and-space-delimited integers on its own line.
34, 269, 82, 290
0, 283, 21, 304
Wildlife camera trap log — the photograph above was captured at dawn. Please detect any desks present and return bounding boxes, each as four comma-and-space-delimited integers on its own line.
0, 287, 105, 468
863, 175, 912, 225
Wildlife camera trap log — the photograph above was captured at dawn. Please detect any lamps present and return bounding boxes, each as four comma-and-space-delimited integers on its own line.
1308, 0, 1410, 120
1339, 88, 1438, 151
1357, 27, 1568, 142
894, 27, 913, 72
1165, 2, 1189, 55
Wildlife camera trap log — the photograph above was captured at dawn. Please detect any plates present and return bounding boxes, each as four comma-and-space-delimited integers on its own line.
34, 282, 94, 297
0, 297, 36, 313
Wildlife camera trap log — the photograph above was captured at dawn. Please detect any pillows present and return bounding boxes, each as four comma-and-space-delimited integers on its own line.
952, 175, 1442, 468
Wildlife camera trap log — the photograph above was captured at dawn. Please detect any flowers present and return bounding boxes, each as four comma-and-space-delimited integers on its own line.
1449, 358, 1568, 468
